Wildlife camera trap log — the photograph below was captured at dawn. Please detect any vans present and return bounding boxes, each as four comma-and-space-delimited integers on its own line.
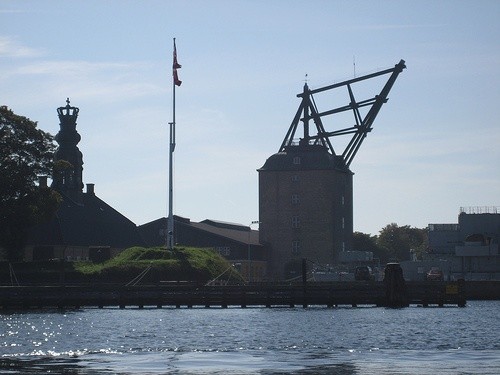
354, 267, 372, 280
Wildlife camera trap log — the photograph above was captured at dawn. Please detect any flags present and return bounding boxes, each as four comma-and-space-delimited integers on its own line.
172, 42, 182, 86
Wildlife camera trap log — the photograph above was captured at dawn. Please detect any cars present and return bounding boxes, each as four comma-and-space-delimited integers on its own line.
426, 267, 443, 279
